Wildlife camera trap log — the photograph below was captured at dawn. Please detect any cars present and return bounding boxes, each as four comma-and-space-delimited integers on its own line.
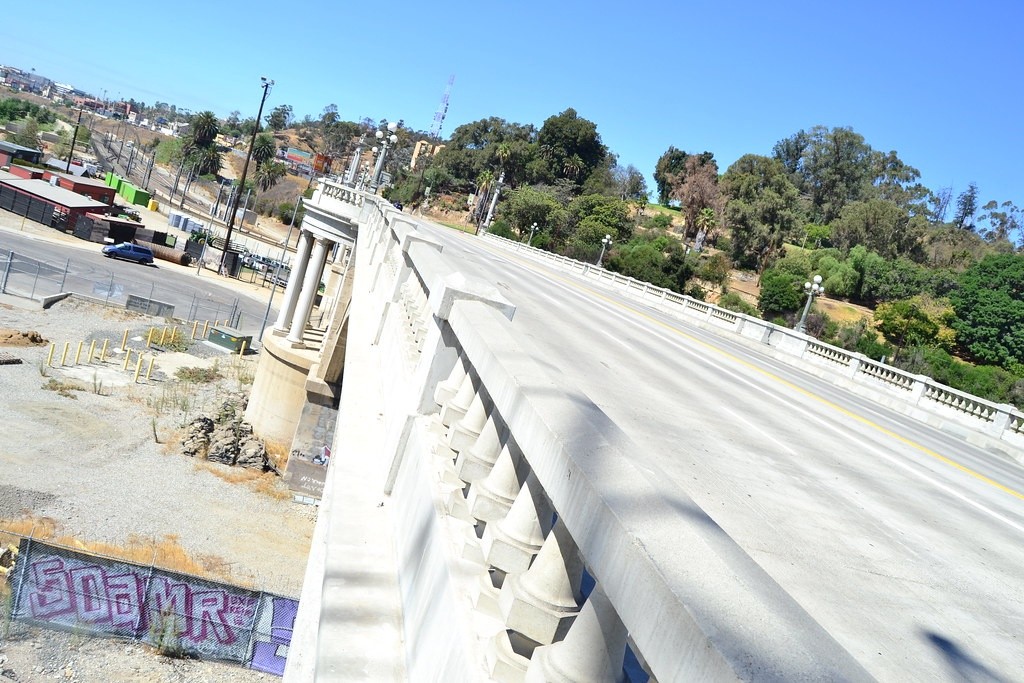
101, 241, 154, 266
393, 203, 403, 210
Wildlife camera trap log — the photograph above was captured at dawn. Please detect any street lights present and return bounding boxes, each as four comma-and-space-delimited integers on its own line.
596, 234, 613, 267
791, 274, 824, 335
368, 122, 398, 194
63, 108, 84, 174
216, 75, 275, 274
527, 222, 539, 245
462, 181, 478, 232
